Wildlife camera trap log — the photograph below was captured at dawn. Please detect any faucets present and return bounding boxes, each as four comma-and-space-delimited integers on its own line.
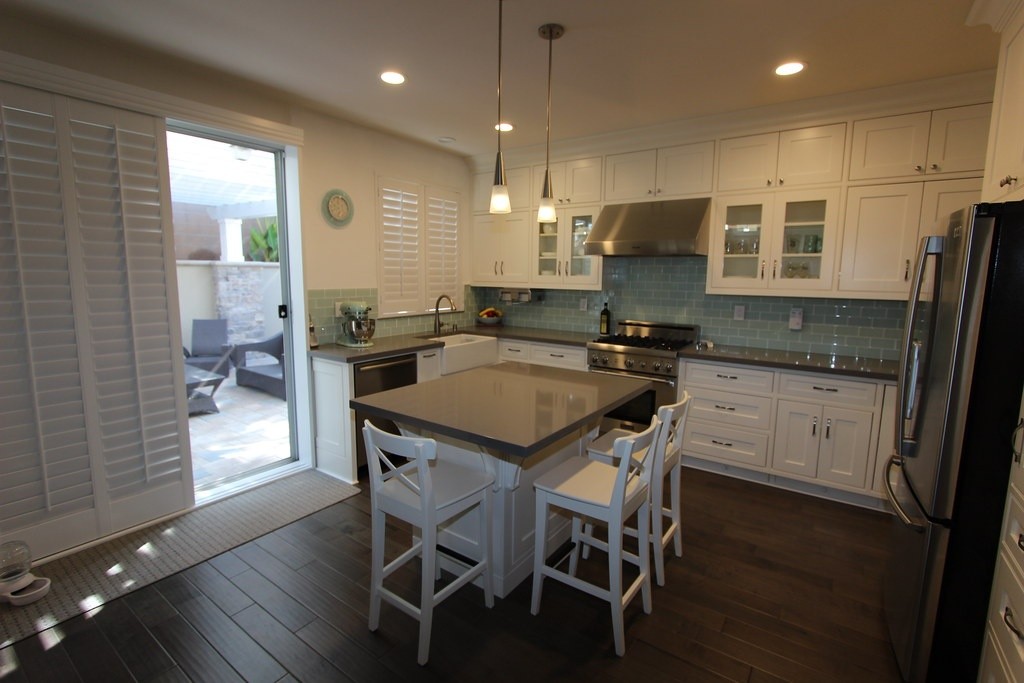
434, 294, 457, 333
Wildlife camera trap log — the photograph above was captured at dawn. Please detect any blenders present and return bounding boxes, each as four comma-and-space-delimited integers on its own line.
335, 300, 377, 347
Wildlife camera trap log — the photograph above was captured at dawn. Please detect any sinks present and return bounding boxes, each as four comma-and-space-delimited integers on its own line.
428, 333, 497, 346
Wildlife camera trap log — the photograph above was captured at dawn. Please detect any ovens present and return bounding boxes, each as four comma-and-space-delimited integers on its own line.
586, 363, 677, 446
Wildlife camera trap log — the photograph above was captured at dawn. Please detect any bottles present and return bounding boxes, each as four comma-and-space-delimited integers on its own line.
599, 303, 610, 334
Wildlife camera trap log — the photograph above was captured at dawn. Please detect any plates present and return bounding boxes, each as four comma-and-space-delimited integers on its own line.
537, 219, 594, 276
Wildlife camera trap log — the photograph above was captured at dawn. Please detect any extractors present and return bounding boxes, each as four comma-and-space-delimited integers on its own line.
584, 197, 712, 257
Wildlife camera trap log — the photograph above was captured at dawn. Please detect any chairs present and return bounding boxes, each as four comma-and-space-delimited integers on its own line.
235, 331, 285, 401
182, 318, 231, 379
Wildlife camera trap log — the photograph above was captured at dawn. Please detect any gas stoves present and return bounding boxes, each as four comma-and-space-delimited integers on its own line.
584, 319, 702, 376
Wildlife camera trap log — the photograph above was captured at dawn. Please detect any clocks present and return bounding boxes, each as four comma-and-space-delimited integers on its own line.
321, 187, 355, 227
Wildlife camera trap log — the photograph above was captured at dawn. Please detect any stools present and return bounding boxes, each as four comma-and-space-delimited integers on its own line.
584, 389, 690, 587
528, 415, 660, 658
362, 417, 498, 667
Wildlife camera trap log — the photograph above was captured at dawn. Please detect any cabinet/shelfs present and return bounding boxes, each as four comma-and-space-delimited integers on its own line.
474, 165, 532, 213
704, 187, 842, 296
500, 340, 586, 368
605, 140, 715, 200
978, 381, 1024, 683
980, 5, 1024, 203
532, 205, 604, 292
772, 370, 875, 491
676, 356, 775, 473
417, 349, 441, 385
873, 381, 901, 502
837, 177, 983, 300
536, 156, 602, 206
470, 211, 530, 283
848, 102, 994, 183
718, 122, 847, 192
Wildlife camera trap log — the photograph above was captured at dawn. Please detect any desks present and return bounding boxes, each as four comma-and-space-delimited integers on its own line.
349, 360, 653, 600
184, 362, 225, 415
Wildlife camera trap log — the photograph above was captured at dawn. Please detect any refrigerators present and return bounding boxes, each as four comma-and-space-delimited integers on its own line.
874, 198, 1024, 682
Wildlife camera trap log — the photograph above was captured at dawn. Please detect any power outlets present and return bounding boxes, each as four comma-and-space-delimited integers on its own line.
335, 302, 344, 317
579, 297, 589, 312
734, 304, 745, 320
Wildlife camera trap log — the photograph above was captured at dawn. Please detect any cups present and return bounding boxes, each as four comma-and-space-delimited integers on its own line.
723, 230, 824, 280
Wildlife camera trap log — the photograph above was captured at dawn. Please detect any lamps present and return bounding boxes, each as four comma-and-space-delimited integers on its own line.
488, 1, 513, 212
537, 23, 565, 224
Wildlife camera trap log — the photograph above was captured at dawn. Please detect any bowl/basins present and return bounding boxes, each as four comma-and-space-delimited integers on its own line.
477, 315, 501, 325
